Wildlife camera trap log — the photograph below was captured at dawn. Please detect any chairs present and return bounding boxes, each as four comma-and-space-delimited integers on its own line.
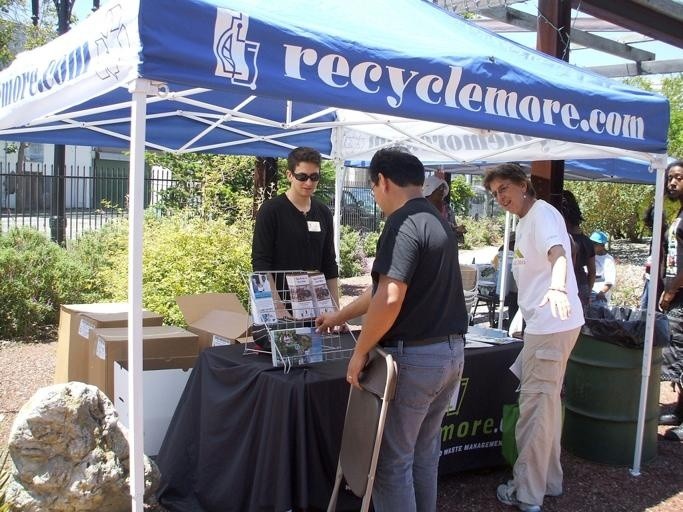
459, 263, 479, 328
326, 344, 400, 512
472, 262, 514, 325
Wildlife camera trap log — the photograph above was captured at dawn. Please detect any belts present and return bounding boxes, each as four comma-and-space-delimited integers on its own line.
386, 334, 458, 347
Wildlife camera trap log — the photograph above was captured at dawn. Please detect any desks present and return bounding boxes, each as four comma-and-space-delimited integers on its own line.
148, 322, 522, 511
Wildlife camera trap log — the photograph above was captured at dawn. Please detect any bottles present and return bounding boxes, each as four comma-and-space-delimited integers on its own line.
496, 250, 513, 297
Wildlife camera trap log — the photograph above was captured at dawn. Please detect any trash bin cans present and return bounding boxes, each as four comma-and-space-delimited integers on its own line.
562, 304, 669, 466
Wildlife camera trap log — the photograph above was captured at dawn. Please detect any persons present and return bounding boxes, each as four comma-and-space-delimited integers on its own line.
490, 191, 614, 308
312, 144, 468, 511
482, 161, 590, 511
421, 172, 467, 247
251, 144, 350, 336
638, 163, 683, 443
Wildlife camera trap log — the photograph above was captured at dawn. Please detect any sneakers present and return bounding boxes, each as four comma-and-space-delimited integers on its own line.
497, 479, 562, 512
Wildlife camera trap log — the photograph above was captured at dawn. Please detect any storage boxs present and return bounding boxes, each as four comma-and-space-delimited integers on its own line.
113, 356, 200, 457
54, 302, 165, 384
88, 324, 200, 405
175, 291, 254, 353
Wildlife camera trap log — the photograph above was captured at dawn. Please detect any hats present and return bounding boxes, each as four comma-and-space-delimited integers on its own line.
424, 176, 449, 198
590, 231, 607, 244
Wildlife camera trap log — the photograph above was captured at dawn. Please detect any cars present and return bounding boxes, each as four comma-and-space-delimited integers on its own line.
313, 187, 383, 233
464, 186, 496, 219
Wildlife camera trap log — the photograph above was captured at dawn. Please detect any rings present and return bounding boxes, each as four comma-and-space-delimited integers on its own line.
348, 375, 353, 379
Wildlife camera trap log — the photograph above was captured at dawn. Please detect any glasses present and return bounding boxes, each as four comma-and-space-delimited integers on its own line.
291, 172, 320, 181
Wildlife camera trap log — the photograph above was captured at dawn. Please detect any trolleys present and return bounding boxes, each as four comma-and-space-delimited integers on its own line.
468, 290, 507, 329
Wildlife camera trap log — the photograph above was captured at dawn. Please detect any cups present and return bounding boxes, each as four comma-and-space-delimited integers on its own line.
477, 281, 496, 296
